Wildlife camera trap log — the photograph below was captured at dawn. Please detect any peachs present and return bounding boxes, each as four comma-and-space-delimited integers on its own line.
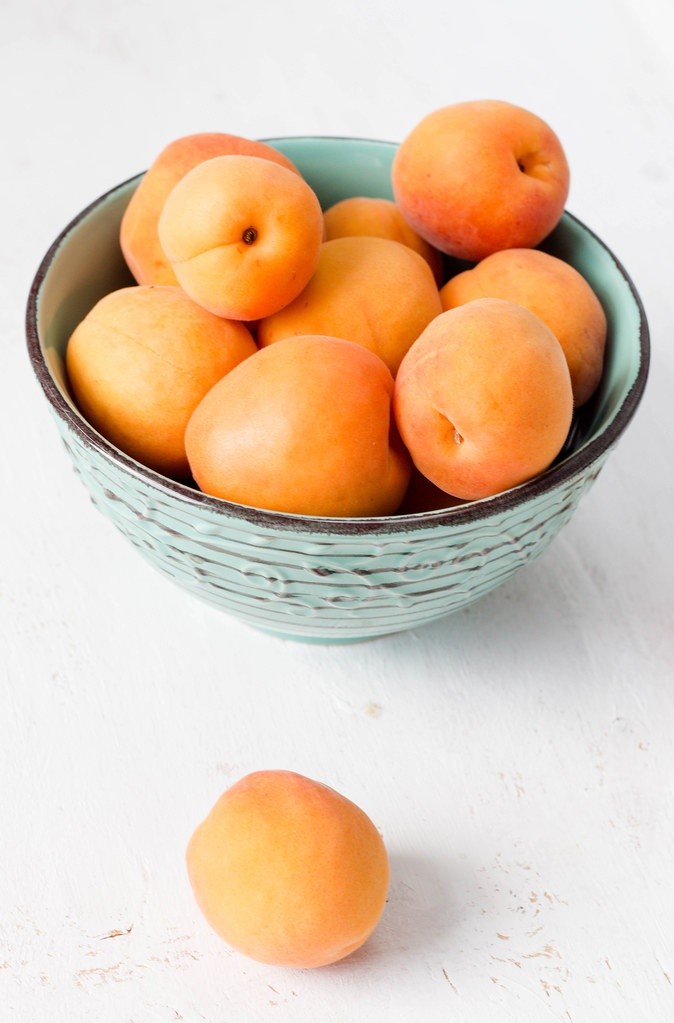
186, 770, 388, 969
68, 97, 606, 516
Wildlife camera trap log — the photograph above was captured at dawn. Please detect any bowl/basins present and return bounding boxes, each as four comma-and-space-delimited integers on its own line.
24, 138, 649, 643
